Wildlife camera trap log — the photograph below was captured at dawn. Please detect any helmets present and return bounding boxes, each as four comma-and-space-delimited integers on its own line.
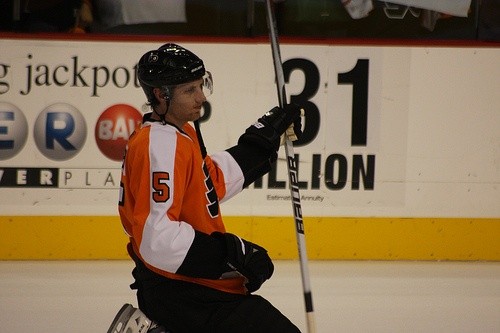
137, 42, 205, 87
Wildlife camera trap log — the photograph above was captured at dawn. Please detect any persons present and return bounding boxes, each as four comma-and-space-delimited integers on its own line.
107, 42, 305, 333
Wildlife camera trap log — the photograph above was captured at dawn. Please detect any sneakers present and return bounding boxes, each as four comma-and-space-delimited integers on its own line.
106, 303, 155, 332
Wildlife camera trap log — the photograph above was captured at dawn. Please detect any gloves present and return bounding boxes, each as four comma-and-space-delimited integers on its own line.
238, 104, 300, 164
212, 231, 273, 292
